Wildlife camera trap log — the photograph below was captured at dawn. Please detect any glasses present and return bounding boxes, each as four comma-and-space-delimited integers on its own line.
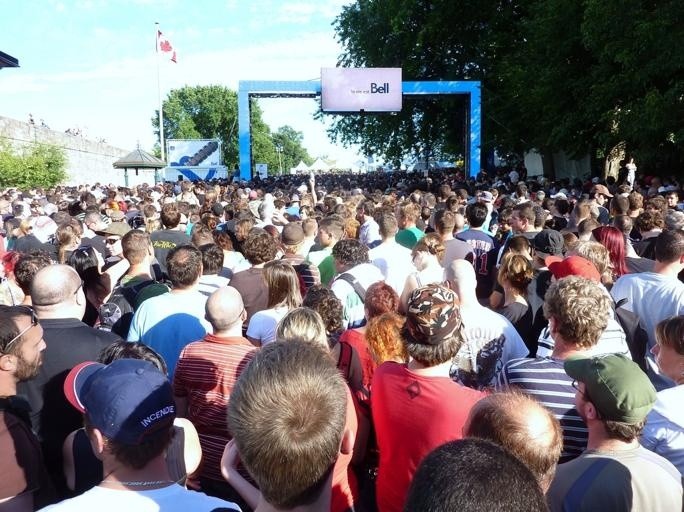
3, 303, 39, 354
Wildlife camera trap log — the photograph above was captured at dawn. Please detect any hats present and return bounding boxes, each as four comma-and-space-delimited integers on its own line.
63, 356, 176, 447
172, 183, 264, 216
562, 352, 659, 424
478, 174, 678, 201
281, 223, 304, 244
402, 284, 460, 344
91, 220, 133, 238
544, 253, 601, 288
532, 229, 565, 261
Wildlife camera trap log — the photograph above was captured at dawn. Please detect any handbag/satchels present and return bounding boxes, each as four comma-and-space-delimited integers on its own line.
614, 299, 648, 360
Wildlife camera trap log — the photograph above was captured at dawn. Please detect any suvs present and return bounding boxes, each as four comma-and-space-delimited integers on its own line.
412, 160, 456, 172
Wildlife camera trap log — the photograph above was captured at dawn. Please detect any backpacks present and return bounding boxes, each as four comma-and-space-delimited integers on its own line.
95, 272, 157, 335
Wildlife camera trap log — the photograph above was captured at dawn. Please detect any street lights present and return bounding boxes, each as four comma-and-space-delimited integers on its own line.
275, 143, 283, 176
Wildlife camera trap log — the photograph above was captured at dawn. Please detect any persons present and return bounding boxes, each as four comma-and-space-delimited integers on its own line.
2, 158, 684, 512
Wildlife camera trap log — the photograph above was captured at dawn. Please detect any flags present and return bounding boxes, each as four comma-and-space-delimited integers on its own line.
156, 29, 177, 63
